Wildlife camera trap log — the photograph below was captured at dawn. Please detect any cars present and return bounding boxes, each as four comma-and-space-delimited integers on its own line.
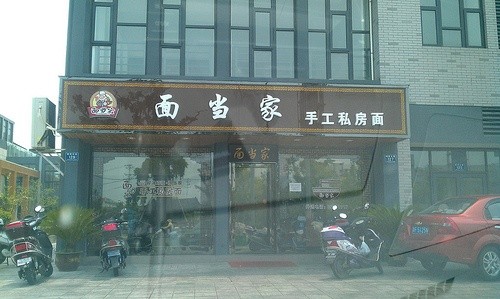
399, 193, 500, 281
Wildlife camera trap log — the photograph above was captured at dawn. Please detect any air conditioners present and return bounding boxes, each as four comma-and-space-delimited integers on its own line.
31, 98, 56, 149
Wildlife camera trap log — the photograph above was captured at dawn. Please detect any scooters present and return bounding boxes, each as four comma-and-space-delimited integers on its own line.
320, 203, 386, 280
0, 205, 54, 285
97, 207, 129, 276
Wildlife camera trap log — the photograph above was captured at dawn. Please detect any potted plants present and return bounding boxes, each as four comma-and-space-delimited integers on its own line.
368, 204, 416, 267
40, 205, 103, 271
